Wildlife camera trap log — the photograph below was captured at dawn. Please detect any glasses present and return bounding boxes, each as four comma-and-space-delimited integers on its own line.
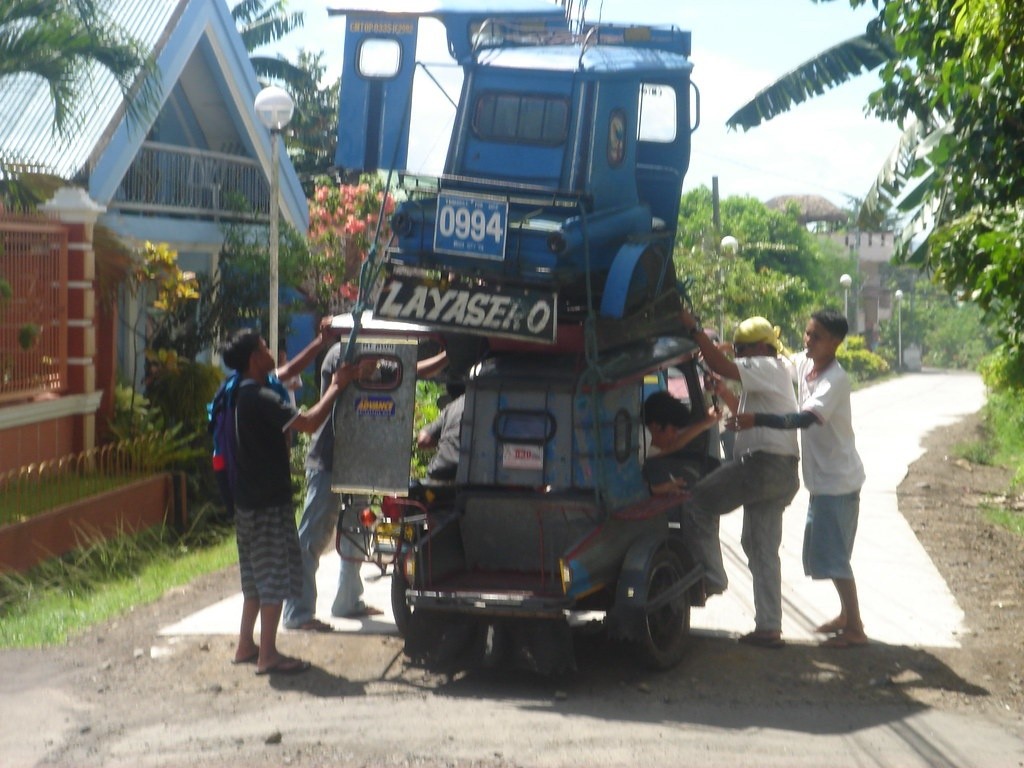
733, 337, 766, 354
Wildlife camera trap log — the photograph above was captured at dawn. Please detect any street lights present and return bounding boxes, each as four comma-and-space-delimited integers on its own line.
894, 289, 905, 372
837, 272, 852, 368
717, 234, 740, 346
253, 83, 297, 373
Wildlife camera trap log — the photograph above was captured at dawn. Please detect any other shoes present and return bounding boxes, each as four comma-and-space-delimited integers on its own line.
294, 618, 332, 633
345, 606, 384, 617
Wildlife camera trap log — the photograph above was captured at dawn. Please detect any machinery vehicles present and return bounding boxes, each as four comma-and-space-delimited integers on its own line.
306, 1, 730, 687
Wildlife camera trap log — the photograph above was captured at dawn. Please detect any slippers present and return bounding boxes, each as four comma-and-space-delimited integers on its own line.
256, 654, 313, 674
817, 616, 845, 631
820, 632, 867, 648
231, 645, 261, 665
738, 632, 785, 649
703, 582, 718, 595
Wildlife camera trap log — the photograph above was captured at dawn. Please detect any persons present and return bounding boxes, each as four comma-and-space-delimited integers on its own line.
679, 305, 800, 649
638, 389, 725, 475
281, 340, 448, 636
416, 391, 466, 483
725, 306, 872, 651
206, 315, 365, 677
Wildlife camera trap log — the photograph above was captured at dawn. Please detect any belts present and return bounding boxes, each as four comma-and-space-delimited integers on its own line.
743, 452, 798, 465
